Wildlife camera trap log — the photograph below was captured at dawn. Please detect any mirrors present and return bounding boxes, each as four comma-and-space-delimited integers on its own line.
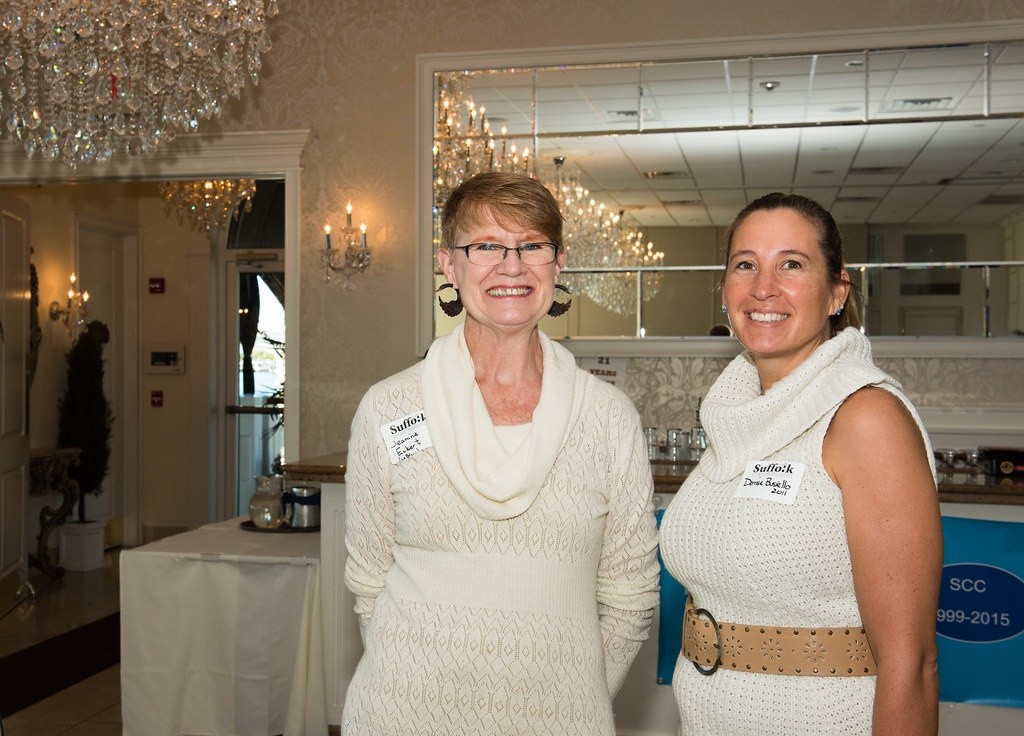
416, 20, 1024, 362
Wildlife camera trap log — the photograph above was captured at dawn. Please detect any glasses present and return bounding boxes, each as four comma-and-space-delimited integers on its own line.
455, 242, 558, 267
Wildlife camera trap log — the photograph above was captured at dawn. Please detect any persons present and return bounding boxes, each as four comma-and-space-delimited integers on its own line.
339, 173, 661, 736
658, 191, 944, 736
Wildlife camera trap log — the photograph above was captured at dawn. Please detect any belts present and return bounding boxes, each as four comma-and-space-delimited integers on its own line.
683, 597, 879, 678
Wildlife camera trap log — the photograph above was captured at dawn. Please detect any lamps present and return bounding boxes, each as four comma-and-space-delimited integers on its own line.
0, 1, 279, 165
49, 271, 89, 327
158, 178, 257, 234
436, 73, 666, 315
324, 200, 370, 289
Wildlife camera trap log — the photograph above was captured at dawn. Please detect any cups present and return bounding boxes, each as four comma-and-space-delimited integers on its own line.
937, 447, 980, 468
286, 485, 321, 528
641, 427, 709, 450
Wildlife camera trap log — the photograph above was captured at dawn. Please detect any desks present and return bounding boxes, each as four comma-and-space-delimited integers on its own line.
119, 517, 331, 736
30, 445, 85, 577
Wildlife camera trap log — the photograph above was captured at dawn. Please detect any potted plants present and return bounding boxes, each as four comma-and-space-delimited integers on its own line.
53, 326, 117, 573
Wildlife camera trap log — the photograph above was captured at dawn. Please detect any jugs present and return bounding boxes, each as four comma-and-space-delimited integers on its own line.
247, 474, 290, 529
